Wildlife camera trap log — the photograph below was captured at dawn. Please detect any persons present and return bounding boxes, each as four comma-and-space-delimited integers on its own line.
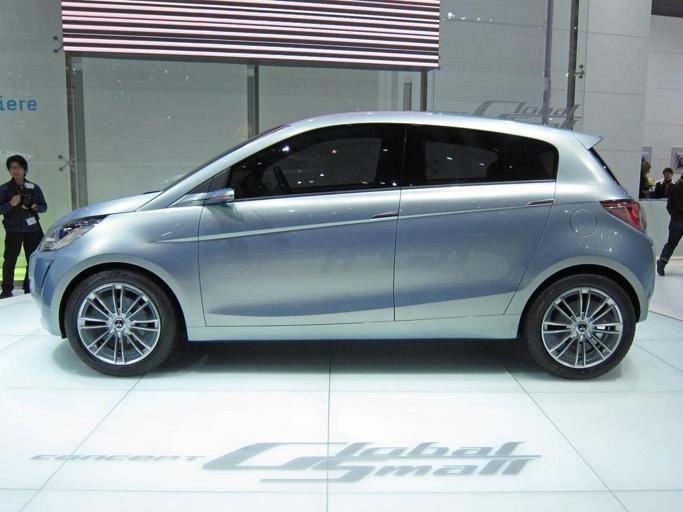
654, 168, 675, 199
656, 170, 683, 276
638, 154, 655, 198
0, 154, 46, 298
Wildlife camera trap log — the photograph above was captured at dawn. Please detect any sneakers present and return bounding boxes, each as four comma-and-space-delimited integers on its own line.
657, 260, 665, 276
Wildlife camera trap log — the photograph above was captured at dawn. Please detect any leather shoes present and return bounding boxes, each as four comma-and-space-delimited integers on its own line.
0, 290, 13, 298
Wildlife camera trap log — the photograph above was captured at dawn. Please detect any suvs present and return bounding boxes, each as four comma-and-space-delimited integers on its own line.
29, 111, 657, 380
